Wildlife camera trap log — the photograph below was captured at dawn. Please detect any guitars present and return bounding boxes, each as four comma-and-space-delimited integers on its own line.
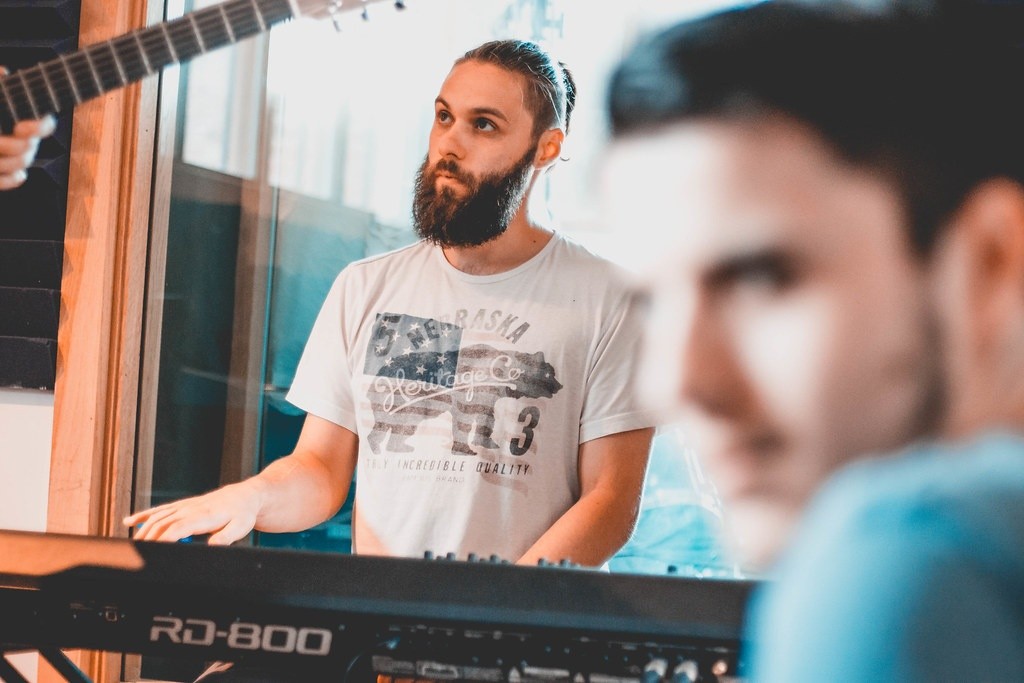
0, 0, 408, 134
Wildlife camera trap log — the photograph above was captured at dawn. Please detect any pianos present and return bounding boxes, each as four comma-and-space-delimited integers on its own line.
0, 526, 763, 683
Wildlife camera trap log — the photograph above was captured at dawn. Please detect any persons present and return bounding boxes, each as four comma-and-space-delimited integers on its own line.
122, 41, 663, 569
606, 0, 1024, 683
